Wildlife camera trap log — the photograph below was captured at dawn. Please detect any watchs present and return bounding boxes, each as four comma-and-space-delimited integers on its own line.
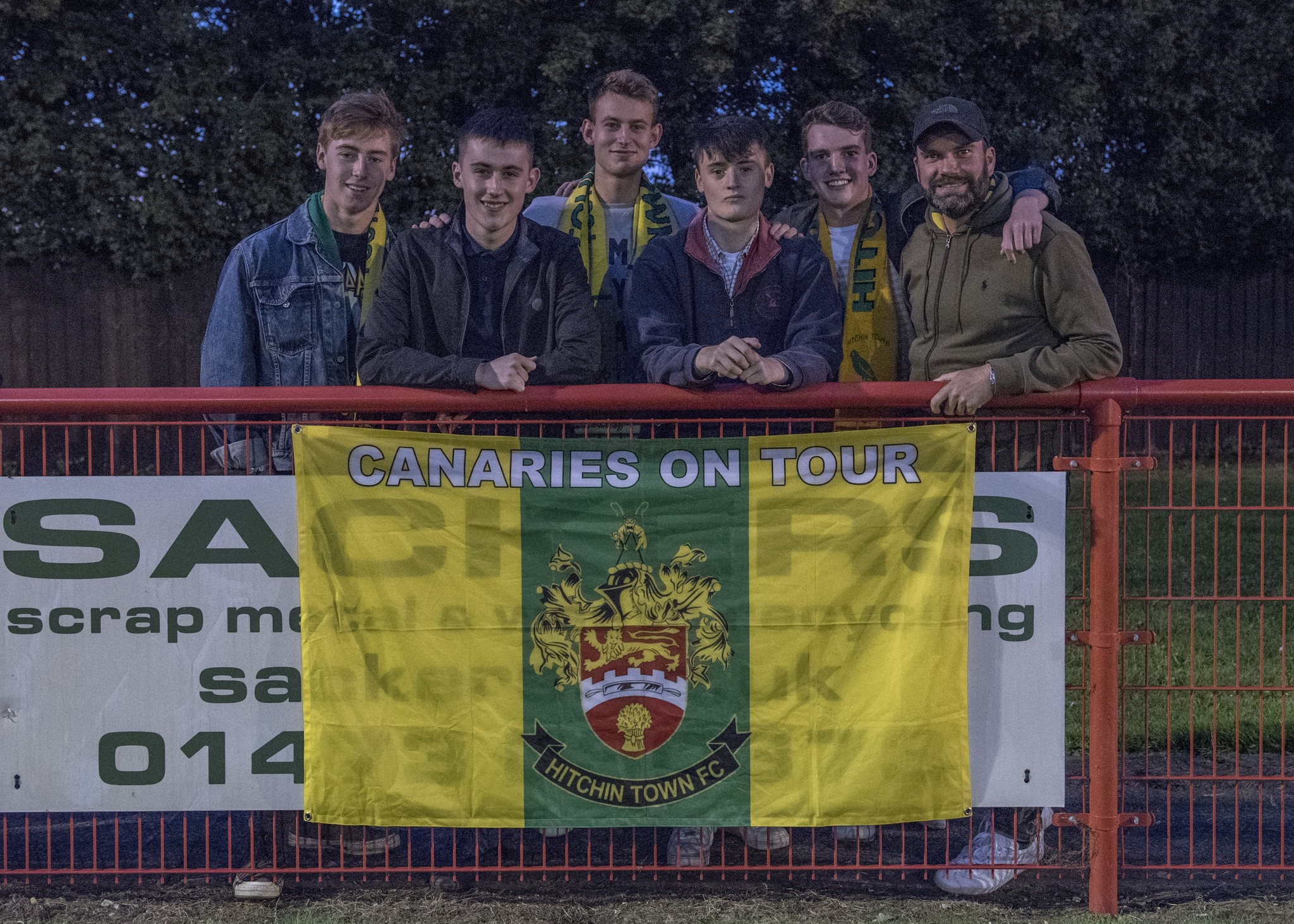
988, 368, 996, 387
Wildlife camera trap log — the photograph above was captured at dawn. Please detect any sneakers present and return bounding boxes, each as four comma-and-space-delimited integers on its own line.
934, 808, 1045, 895
288, 821, 401, 856
721, 826, 790, 851
667, 826, 719, 867
233, 845, 285, 898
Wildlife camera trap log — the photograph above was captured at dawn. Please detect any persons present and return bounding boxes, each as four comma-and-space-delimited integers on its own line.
778, 97, 1059, 849
631, 115, 844, 878
357, 108, 607, 893
410, 68, 808, 837
199, 88, 403, 899
892, 97, 1127, 893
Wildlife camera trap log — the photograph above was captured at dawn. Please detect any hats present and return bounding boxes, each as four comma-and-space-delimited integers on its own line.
911, 96, 991, 147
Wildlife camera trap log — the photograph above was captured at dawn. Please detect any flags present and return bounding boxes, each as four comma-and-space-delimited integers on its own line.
294, 422, 976, 827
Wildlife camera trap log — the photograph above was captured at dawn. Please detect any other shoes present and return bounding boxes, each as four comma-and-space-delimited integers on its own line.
919, 819, 946, 830
832, 825, 875, 842
